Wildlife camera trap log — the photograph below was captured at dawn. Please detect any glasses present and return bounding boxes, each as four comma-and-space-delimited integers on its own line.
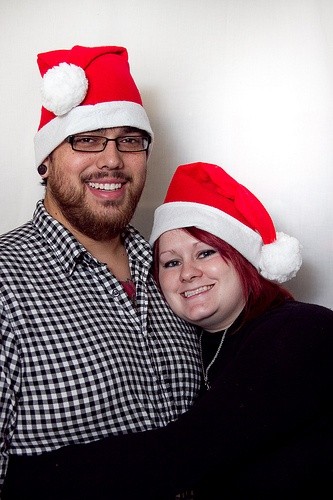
65, 135, 151, 152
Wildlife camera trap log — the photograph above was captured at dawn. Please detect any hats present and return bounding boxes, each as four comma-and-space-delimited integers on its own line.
34, 45, 154, 170
149, 162, 303, 284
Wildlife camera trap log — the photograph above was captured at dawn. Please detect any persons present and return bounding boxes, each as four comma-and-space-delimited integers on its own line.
0, 45, 205, 500
0, 163, 333, 500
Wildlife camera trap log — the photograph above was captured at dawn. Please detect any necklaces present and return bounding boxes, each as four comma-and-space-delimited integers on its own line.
199, 329, 227, 390
